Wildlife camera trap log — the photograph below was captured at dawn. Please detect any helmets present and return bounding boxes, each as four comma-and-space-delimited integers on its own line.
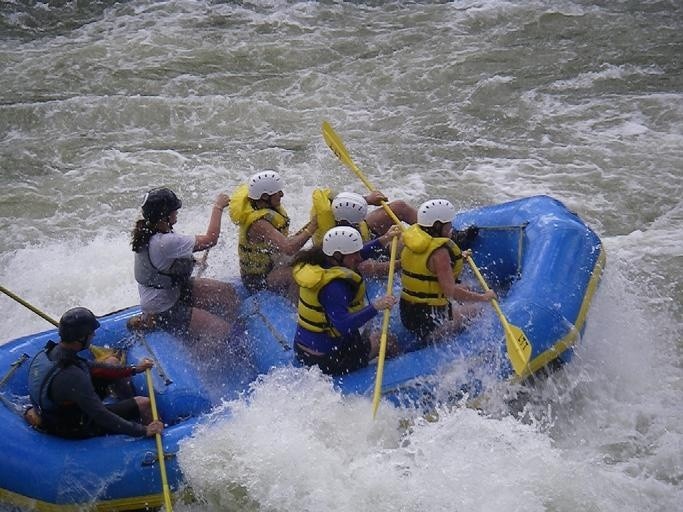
322, 192, 367, 256
416, 199, 456, 227
59, 307, 100, 339
247, 170, 283, 200
142, 187, 181, 222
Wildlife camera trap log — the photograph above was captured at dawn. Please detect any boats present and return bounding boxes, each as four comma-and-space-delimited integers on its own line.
0, 194, 606, 512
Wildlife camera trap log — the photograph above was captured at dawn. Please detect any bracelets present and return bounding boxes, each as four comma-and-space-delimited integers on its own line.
212, 204, 224, 212
129, 362, 137, 377
305, 228, 312, 237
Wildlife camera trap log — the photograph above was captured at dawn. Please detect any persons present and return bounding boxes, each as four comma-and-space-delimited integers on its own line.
27, 306, 168, 440
131, 188, 235, 361
289, 226, 402, 378
310, 189, 418, 278
229, 170, 319, 302
399, 199, 497, 346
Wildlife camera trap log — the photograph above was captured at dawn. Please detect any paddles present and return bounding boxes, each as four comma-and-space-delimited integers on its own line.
194, 200, 229, 281
1, 287, 117, 365
466, 248, 531, 375
323, 120, 408, 233
